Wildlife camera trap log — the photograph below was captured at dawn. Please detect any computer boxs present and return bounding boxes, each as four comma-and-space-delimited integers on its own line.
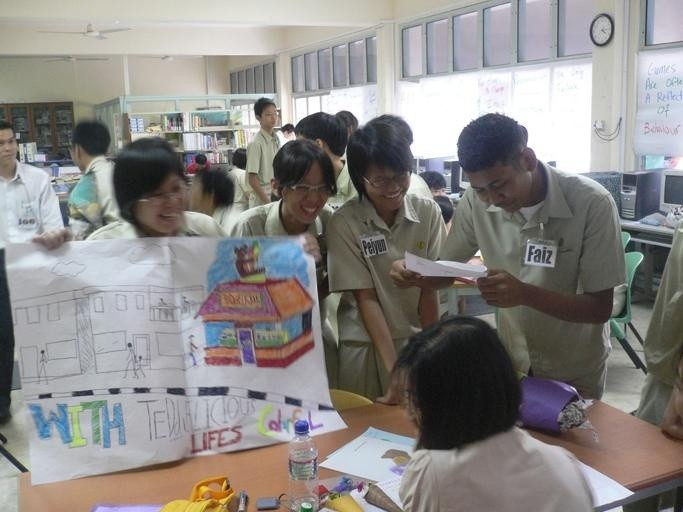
619, 169, 660, 221
443, 160, 460, 195
417, 158, 426, 176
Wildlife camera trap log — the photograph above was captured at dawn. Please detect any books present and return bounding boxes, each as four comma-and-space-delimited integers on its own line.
161, 115, 232, 164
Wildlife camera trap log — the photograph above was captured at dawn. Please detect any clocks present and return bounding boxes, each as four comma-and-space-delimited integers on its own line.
587, 11, 614, 48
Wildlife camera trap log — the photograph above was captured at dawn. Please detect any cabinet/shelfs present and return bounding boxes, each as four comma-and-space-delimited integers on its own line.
123, 109, 253, 165
0, 103, 73, 153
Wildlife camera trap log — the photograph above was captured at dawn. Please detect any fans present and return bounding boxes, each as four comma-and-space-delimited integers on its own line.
39, 54, 113, 66
33, 20, 134, 45
138, 55, 204, 65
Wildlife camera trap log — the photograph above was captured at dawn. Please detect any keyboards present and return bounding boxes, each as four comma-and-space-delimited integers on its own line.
631, 232, 673, 244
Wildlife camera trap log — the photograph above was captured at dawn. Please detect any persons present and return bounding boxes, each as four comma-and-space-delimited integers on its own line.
187, 334, 200, 368
1, 122, 63, 242
32, 135, 223, 248
187, 148, 250, 235
385, 313, 597, 512
158, 297, 169, 318
245, 97, 281, 207
123, 342, 138, 379
323, 118, 448, 408
225, 137, 341, 391
277, 110, 358, 210
35, 350, 50, 385
383, 114, 453, 227
183, 296, 190, 313
386, 112, 626, 403
631, 219, 681, 443
134, 356, 146, 378
68, 120, 120, 240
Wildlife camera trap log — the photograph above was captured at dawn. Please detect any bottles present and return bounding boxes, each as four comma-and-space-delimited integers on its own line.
288, 420, 319, 512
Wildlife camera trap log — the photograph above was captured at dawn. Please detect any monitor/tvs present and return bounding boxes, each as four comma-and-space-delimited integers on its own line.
459, 165, 470, 194
659, 169, 683, 213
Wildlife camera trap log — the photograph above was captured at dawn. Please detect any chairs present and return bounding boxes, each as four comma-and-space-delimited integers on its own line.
619, 231, 631, 252
608, 251, 649, 379
315, 385, 373, 414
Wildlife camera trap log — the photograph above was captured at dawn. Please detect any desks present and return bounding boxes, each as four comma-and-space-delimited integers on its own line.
15, 378, 681, 512
618, 213, 683, 302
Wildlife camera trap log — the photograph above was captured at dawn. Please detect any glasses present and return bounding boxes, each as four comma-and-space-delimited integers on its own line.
138, 179, 185, 204
290, 184, 331, 194
362, 169, 412, 187
